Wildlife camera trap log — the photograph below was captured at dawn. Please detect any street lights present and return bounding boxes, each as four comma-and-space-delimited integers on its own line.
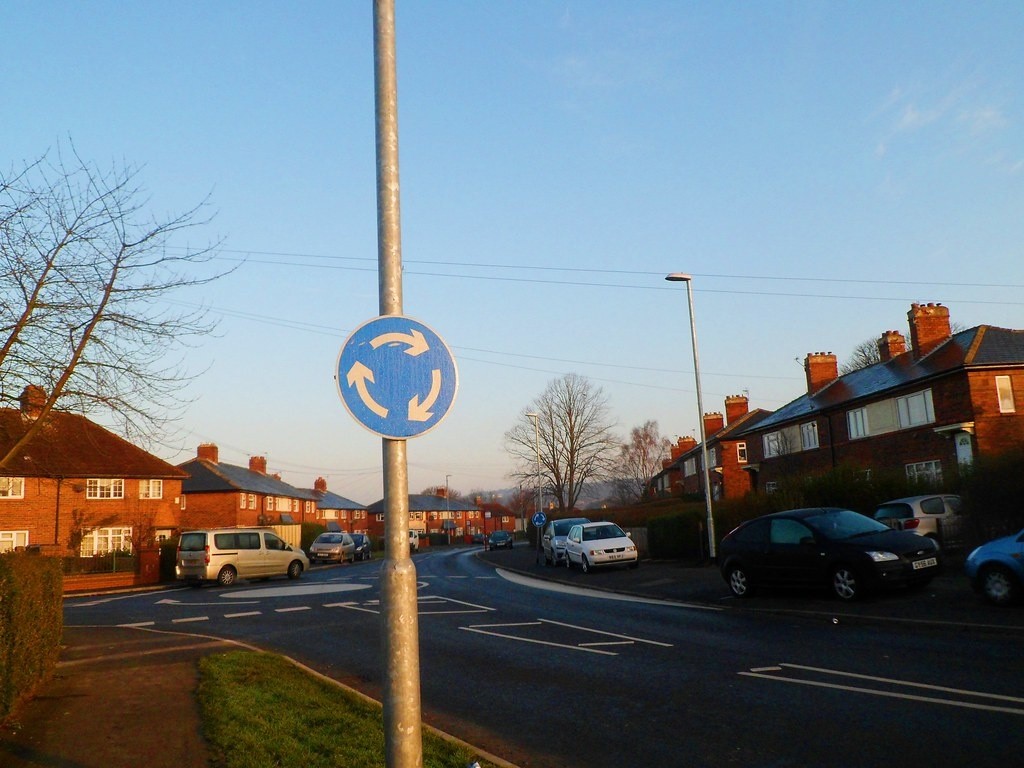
664, 273, 717, 556
524, 414, 542, 565
445, 475, 453, 545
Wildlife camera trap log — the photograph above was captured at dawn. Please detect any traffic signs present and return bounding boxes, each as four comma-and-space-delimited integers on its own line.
335, 314, 459, 439
531, 512, 547, 527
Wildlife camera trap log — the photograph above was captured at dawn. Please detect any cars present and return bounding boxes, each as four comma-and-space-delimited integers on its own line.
472, 533, 485, 544
488, 531, 512, 550
349, 534, 370, 560
409, 530, 419, 553
714, 507, 938, 602
966, 530, 1024, 606
310, 532, 355, 564
565, 521, 638, 573
541, 518, 593, 567
872, 494, 964, 551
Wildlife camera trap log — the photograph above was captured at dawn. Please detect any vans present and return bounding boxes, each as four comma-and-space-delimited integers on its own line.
174, 528, 310, 587
